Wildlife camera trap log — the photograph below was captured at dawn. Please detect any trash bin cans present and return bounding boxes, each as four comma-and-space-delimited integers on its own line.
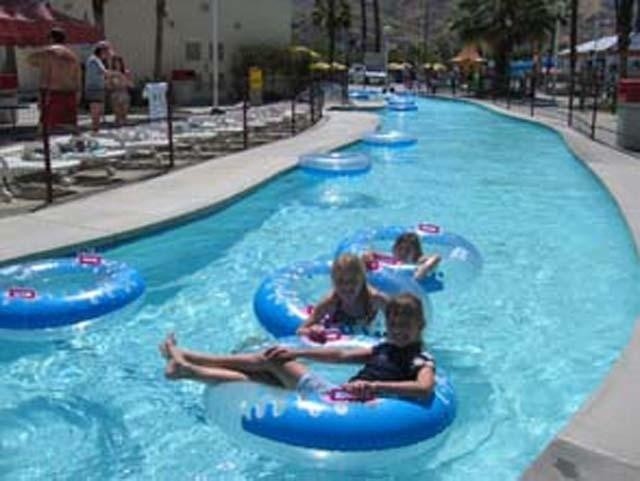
617, 78, 640, 151
145, 82, 167, 119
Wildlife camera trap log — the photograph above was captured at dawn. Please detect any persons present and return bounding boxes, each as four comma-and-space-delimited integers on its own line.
104, 55, 135, 123
35, 26, 83, 140
363, 234, 442, 282
296, 251, 390, 343
160, 290, 437, 399
401, 63, 476, 97
84, 40, 124, 136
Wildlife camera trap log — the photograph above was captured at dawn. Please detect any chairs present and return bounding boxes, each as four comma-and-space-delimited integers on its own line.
1, 111, 242, 200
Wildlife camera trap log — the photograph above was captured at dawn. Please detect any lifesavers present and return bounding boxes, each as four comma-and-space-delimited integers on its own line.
361, 129, 417, 149
1, 255, 146, 343
204, 334, 460, 467
253, 251, 434, 334
299, 150, 370, 176
389, 97, 417, 111
336, 222, 480, 291
